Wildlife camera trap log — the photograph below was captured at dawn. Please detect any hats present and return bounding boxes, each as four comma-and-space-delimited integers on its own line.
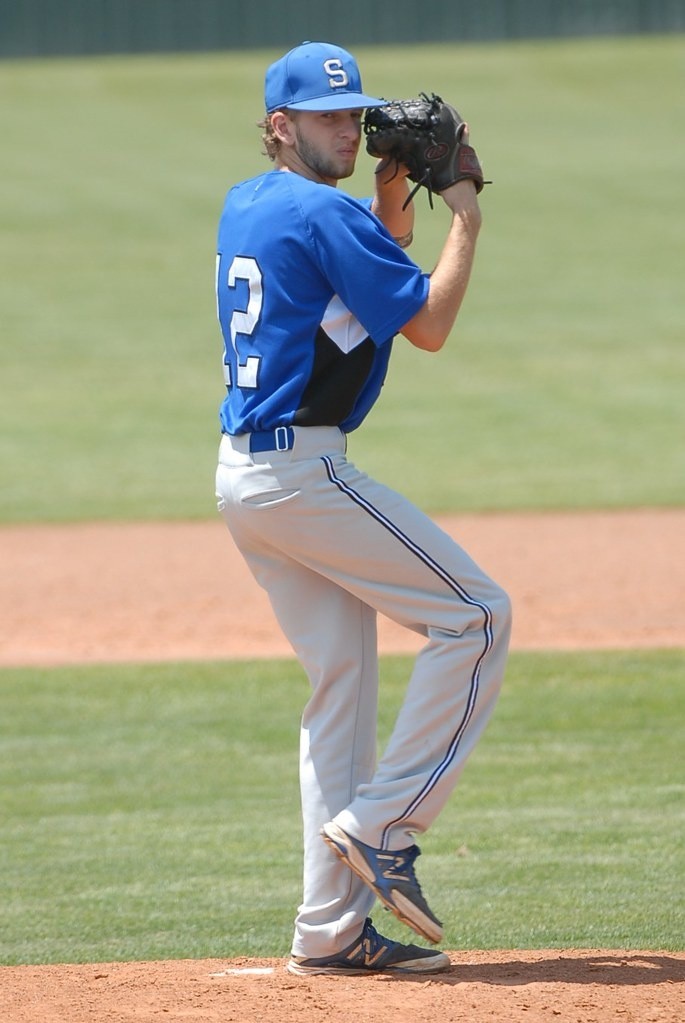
265, 40, 391, 113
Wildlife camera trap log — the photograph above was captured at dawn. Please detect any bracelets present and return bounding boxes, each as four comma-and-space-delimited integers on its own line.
391, 229, 413, 248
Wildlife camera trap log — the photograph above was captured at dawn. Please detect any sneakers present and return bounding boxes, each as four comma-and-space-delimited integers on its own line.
286, 920, 452, 976
319, 822, 443, 945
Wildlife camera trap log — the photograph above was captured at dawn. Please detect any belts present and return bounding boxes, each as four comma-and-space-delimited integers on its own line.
249, 426, 295, 455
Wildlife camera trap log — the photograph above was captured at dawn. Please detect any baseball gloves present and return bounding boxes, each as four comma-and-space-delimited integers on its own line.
360, 93, 493, 211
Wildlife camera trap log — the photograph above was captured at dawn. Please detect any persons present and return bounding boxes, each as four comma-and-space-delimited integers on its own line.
212, 39, 513, 977
